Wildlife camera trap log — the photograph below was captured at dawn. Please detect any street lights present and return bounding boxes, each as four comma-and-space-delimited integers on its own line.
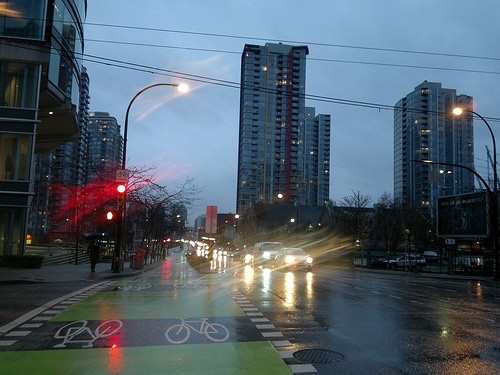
113, 82, 190, 270
453, 106, 500, 282
277, 193, 299, 247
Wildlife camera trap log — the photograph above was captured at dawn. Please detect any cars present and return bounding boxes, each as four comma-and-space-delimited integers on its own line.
370, 249, 494, 278
274, 246, 314, 271
177, 238, 254, 267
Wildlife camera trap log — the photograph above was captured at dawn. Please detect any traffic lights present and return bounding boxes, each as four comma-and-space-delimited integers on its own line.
115, 180, 126, 201
105, 209, 115, 222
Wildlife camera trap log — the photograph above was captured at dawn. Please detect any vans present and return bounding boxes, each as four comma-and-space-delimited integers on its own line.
252, 241, 283, 266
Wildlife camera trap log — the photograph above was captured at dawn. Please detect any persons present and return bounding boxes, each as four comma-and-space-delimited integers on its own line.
89, 239, 100, 272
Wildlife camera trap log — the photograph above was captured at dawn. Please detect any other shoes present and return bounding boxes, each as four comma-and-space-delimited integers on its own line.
91, 269, 95, 272
